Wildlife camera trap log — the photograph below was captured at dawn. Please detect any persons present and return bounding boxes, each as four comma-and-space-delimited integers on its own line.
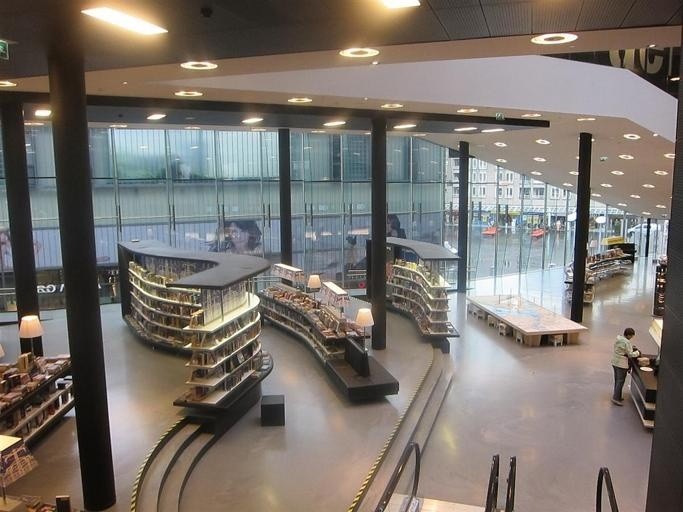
611, 327, 641, 407
208, 222, 262, 258
341, 213, 408, 273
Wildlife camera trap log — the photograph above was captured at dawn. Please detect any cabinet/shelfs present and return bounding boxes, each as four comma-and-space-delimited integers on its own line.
118, 238, 275, 436
564, 252, 634, 305
0, 356, 75, 445
385, 235, 461, 352
257, 290, 369, 369
625, 347, 663, 430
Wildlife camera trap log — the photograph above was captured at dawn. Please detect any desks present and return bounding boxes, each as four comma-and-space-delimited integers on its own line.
466, 293, 587, 347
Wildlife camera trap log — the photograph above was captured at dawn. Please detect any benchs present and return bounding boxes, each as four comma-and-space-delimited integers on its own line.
324, 336, 400, 402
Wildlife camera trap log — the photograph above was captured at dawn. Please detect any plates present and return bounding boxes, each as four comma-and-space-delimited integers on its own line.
639, 366, 653, 372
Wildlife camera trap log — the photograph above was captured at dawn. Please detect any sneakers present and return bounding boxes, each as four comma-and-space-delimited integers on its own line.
611, 398, 624, 407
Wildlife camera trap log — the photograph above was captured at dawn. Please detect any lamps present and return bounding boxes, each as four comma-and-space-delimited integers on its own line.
18, 315, 44, 362
306, 273, 322, 302
0, 434, 38, 512
355, 308, 374, 347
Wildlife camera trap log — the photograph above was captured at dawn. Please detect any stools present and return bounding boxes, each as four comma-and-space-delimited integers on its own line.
467, 302, 511, 337
260, 393, 285, 428
515, 329, 527, 343
546, 333, 563, 347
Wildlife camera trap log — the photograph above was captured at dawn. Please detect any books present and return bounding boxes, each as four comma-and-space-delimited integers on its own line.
565, 246, 632, 305
2, 492, 58, 511
252, 263, 373, 365
653, 254, 667, 314
116, 260, 263, 400
384, 258, 459, 339
0, 351, 74, 446
